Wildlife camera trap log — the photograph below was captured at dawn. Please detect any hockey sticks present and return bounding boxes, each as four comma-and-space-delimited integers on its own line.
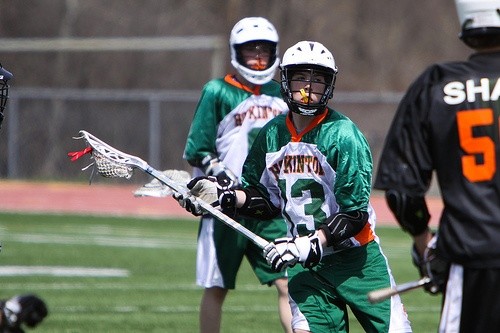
78, 130, 269, 251
367, 276, 431, 305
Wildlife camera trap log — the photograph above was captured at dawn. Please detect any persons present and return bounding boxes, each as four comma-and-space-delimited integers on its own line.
171, 40, 413, 332
371, 0, 500, 332
181, 16, 294, 332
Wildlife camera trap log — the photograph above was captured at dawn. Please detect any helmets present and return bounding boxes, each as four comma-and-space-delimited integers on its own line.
229, 17, 281, 85
279, 41, 338, 115
454, 1, 500, 49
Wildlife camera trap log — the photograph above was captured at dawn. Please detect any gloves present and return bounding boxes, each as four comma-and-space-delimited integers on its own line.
411, 233, 448, 295
202, 155, 239, 187
172, 175, 238, 217
261, 230, 322, 273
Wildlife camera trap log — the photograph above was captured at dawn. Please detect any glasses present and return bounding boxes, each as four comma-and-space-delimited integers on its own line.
235, 45, 275, 57
